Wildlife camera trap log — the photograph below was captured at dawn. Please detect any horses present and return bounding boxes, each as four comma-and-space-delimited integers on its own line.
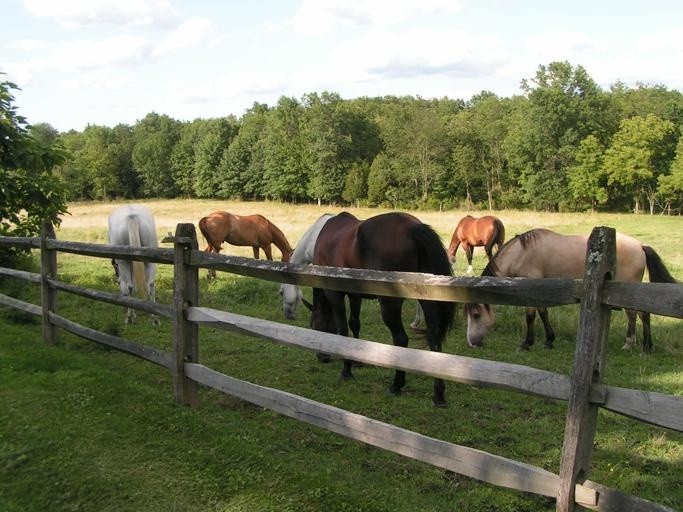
300, 211, 458, 408
279, 212, 424, 331
448, 215, 505, 273
198, 210, 295, 280
106, 203, 162, 327
463, 229, 683, 353
